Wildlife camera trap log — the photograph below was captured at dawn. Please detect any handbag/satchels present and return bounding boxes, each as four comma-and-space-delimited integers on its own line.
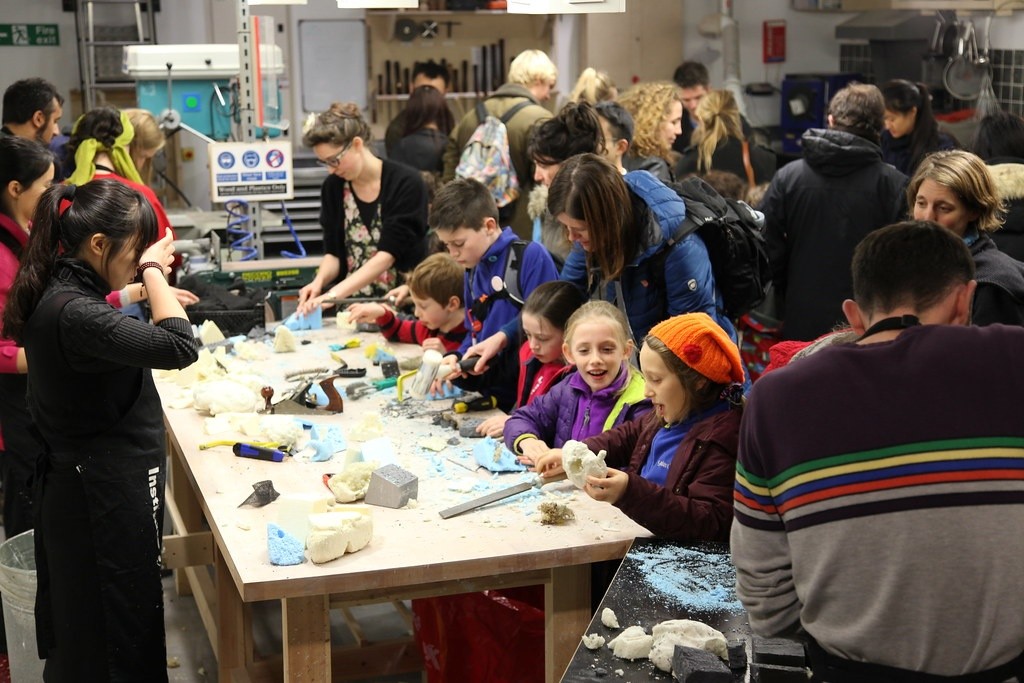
411, 588, 546, 683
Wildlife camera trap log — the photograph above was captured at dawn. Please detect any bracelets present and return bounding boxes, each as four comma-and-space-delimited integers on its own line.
140, 261, 164, 297
325, 290, 336, 299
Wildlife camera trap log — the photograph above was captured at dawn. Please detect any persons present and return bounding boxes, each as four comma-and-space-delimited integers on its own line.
3, 179, 200, 683
730, 220, 1024, 683
296, 48, 778, 552
756, 78, 1024, 341
0, 77, 174, 654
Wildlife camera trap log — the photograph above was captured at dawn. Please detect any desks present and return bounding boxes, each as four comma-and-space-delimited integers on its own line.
145, 316, 655, 683
559, 537, 753, 683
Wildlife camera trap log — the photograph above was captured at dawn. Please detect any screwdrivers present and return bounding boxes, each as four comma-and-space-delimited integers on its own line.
408, 396, 497, 419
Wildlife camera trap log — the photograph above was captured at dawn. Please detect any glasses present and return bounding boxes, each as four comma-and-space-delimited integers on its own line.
316, 139, 351, 168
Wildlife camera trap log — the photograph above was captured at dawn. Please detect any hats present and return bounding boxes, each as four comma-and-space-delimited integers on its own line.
649, 311, 745, 387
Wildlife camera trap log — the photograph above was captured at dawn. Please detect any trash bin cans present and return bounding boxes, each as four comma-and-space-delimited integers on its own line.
0, 528, 45, 683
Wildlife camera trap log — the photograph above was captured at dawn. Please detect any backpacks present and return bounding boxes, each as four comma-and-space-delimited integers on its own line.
455, 99, 539, 223
650, 175, 772, 328
